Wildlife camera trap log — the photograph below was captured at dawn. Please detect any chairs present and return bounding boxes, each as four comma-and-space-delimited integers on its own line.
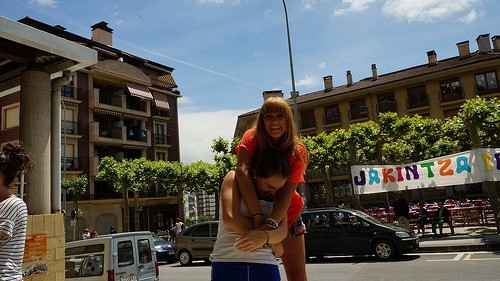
368, 199, 497, 227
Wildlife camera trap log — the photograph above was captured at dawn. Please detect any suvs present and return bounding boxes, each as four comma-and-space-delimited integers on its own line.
301, 206, 422, 262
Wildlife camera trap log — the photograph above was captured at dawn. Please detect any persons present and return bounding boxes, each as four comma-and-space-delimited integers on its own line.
436, 201, 455, 237
235, 97, 309, 281
394, 193, 410, 230
110, 225, 117, 234
0, 140, 36, 281
168, 219, 175, 242
416, 202, 431, 234
175, 218, 182, 239
209, 151, 291, 281
80, 227, 99, 239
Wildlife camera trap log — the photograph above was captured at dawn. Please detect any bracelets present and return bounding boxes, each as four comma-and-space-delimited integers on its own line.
264, 230, 271, 246
266, 216, 280, 229
252, 209, 266, 218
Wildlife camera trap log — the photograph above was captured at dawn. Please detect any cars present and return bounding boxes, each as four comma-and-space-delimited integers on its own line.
174, 220, 220, 267
137, 236, 175, 264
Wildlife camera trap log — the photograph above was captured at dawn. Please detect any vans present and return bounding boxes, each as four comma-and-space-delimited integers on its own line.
65, 230, 160, 281
